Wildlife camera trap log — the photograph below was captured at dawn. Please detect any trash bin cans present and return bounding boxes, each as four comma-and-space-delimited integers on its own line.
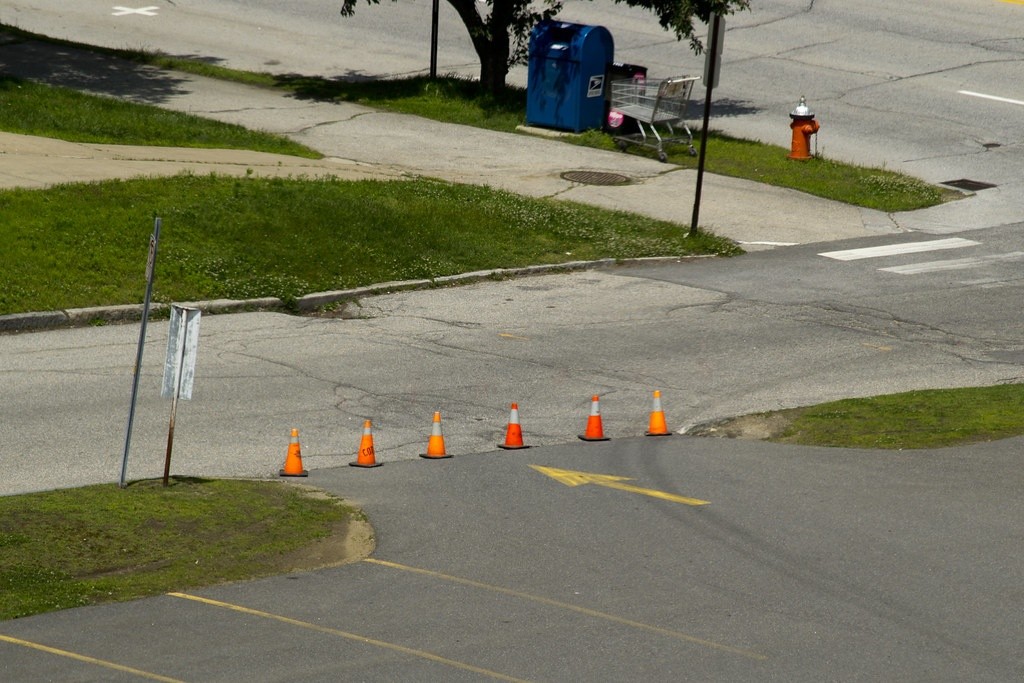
526, 18, 614, 133
602, 62, 648, 134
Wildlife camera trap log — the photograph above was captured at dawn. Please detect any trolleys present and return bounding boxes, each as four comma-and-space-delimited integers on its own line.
610, 74, 702, 163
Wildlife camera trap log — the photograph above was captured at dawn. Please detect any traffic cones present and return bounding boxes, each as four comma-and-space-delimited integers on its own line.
279, 428, 308, 477
419, 411, 454, 459
496, 402, 532, 450
577, 395, 612, 442
644, 390, 672, 436
348, 420, 383, 468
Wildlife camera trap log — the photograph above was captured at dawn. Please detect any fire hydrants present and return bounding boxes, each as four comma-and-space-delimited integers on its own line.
786, 95, 820, 161
